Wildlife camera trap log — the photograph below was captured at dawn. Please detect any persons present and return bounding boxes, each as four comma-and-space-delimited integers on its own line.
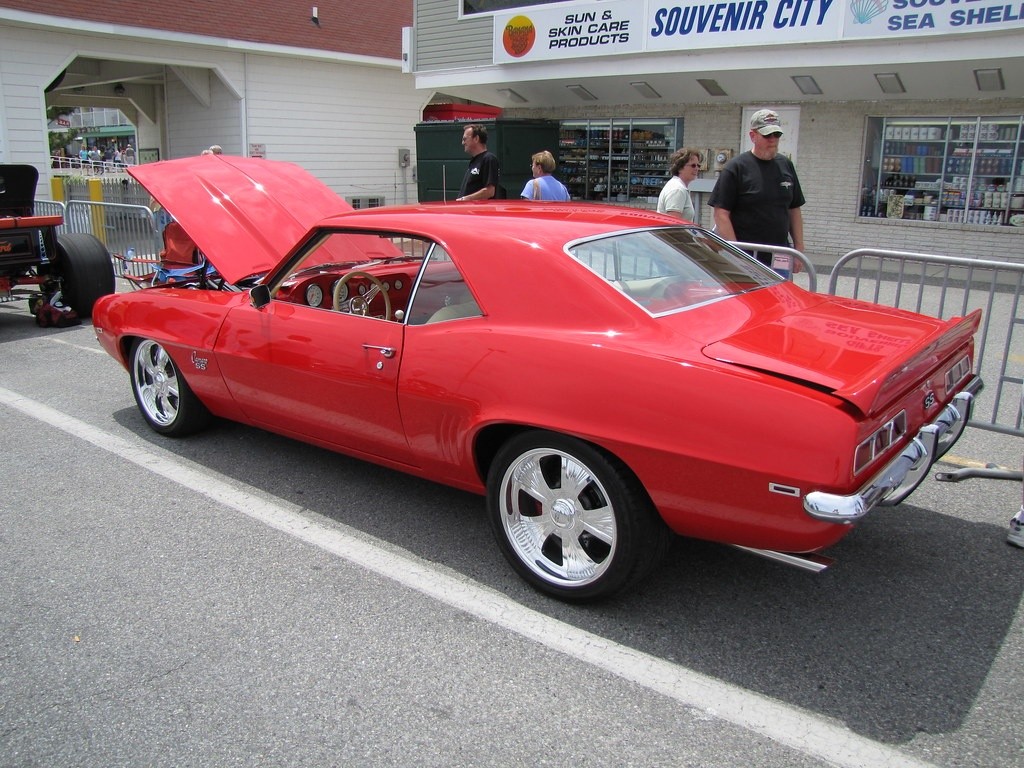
708, 109, 805, 285
456, 123, 500, 201
519, 151, 571, 201
652, 146, 702, 281
79, 143, 135, 176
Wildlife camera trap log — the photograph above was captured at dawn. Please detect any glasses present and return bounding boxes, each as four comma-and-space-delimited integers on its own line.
685, 163, 701, 169
530, 164, 539, 167
763, 131, 784, 139
462, 137, 473, 142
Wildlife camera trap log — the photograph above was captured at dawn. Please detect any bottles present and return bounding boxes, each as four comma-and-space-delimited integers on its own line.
923, 196, 932, 205
882, 157, 942, 173
884, 141, 945, 156
986, 211, 1004, 225
879, 189, 896, 204
951, 142, 973, 156
914, 198, 923, 203
999, 127, 1018, 141
942, 191, 966, 207
883, 175, 916, 188
904, 195, 914, 206
886, 126, 941, 141
972, 159, 1011, 175
971, 192, 1009, 208
945, 158, 971, 175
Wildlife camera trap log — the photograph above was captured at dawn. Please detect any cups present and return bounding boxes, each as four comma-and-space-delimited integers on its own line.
960, 125, 976, 141
904, 212, 923, 220
968, 210, 986, 224
916, 179, 941, 189
979, 125, 998, 141
944, 176, 968, 190
972, 178, 1005, 192
947, 209, 964, 223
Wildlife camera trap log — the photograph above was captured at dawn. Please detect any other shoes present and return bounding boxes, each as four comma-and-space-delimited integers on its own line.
1007, 511, 1024, 547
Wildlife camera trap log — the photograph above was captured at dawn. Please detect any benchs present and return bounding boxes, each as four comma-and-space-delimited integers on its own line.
422, 274, 690, 329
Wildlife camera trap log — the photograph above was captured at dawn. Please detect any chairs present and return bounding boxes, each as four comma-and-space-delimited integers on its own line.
111, 221, 205, 290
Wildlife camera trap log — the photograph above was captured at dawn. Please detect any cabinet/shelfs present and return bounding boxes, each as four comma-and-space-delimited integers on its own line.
875, 117, 1024, 227
558, 118, 677, 201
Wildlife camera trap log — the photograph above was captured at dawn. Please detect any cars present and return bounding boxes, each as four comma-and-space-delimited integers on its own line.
91, 152, 986, 607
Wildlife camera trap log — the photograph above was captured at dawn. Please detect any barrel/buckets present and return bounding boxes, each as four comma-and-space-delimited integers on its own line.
1010, 197, 1024, 208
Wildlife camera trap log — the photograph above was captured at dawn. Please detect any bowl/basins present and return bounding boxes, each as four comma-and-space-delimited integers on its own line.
931, 199, 938, 204
923, 206, 937, 221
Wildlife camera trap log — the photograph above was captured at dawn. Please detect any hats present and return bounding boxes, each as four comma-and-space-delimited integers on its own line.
750, 109, 784, 136
